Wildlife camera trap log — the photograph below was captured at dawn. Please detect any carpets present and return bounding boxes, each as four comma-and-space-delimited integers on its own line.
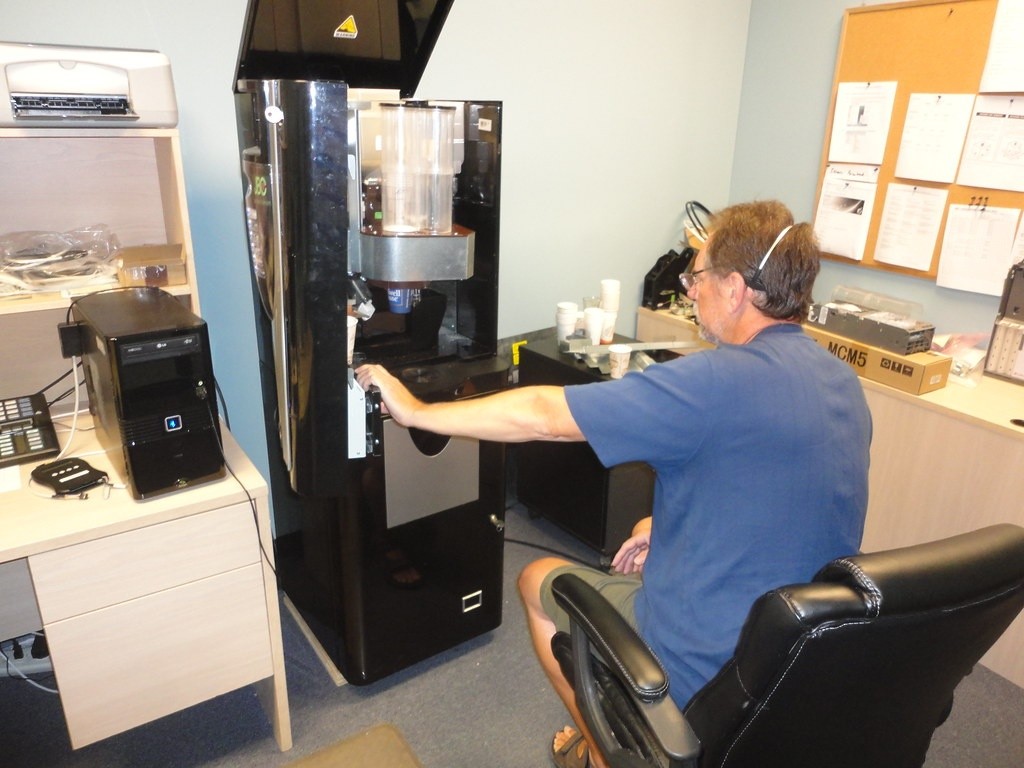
271, 722, 422, 768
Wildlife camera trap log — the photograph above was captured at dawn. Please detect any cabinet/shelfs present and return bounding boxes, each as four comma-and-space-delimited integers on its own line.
635, 304, 1024, 692
0, 128, 294, 754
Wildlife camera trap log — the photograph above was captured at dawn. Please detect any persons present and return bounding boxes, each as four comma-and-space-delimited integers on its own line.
356, 198, 873, 768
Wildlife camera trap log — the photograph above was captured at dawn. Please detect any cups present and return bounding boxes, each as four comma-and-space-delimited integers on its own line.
608, 344, 632, 380
555, 278, 621, 345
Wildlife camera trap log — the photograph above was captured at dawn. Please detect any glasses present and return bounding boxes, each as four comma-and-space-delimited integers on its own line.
678, 259, 740, 289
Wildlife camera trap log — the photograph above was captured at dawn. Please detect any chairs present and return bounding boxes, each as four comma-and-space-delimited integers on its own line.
551, 522, 1024, 768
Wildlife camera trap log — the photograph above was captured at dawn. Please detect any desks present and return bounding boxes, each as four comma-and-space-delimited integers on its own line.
516, 331, 690, 558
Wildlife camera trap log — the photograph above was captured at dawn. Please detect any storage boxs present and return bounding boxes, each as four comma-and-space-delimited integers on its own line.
115, 242, 187, 287
801, 325, 953, 396
807, 299, 937, 356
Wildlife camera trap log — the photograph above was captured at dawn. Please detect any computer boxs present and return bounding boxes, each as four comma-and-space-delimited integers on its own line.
70, 289, 226, 501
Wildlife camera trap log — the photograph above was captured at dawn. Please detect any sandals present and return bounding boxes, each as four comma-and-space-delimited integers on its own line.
548, 730, 595, 768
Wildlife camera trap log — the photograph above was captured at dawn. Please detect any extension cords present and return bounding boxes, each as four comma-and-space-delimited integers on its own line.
0, 648, 53, 678
